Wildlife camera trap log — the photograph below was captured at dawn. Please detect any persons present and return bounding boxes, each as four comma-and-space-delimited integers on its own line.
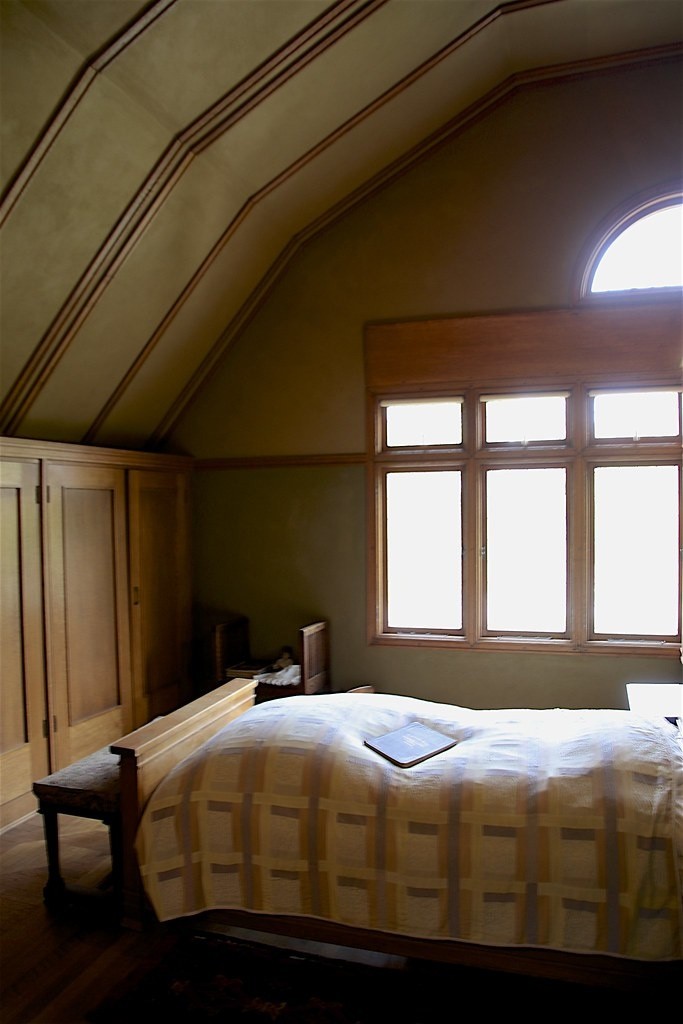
259, 649, 293, 674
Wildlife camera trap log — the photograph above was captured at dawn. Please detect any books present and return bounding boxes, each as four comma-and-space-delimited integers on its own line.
363, 721, 458, 768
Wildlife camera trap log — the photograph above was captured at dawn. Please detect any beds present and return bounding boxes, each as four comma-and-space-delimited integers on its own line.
109, 674, 683, 1002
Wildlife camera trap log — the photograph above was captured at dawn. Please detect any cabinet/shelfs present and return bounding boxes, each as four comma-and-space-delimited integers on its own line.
0, 437, 198, 831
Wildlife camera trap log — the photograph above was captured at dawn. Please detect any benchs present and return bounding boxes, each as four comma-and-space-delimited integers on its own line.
31, 716, 168, 930
212, 614, 330, 702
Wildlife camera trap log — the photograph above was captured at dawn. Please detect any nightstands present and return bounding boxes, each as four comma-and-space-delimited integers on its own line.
626, 683, 683, 724
